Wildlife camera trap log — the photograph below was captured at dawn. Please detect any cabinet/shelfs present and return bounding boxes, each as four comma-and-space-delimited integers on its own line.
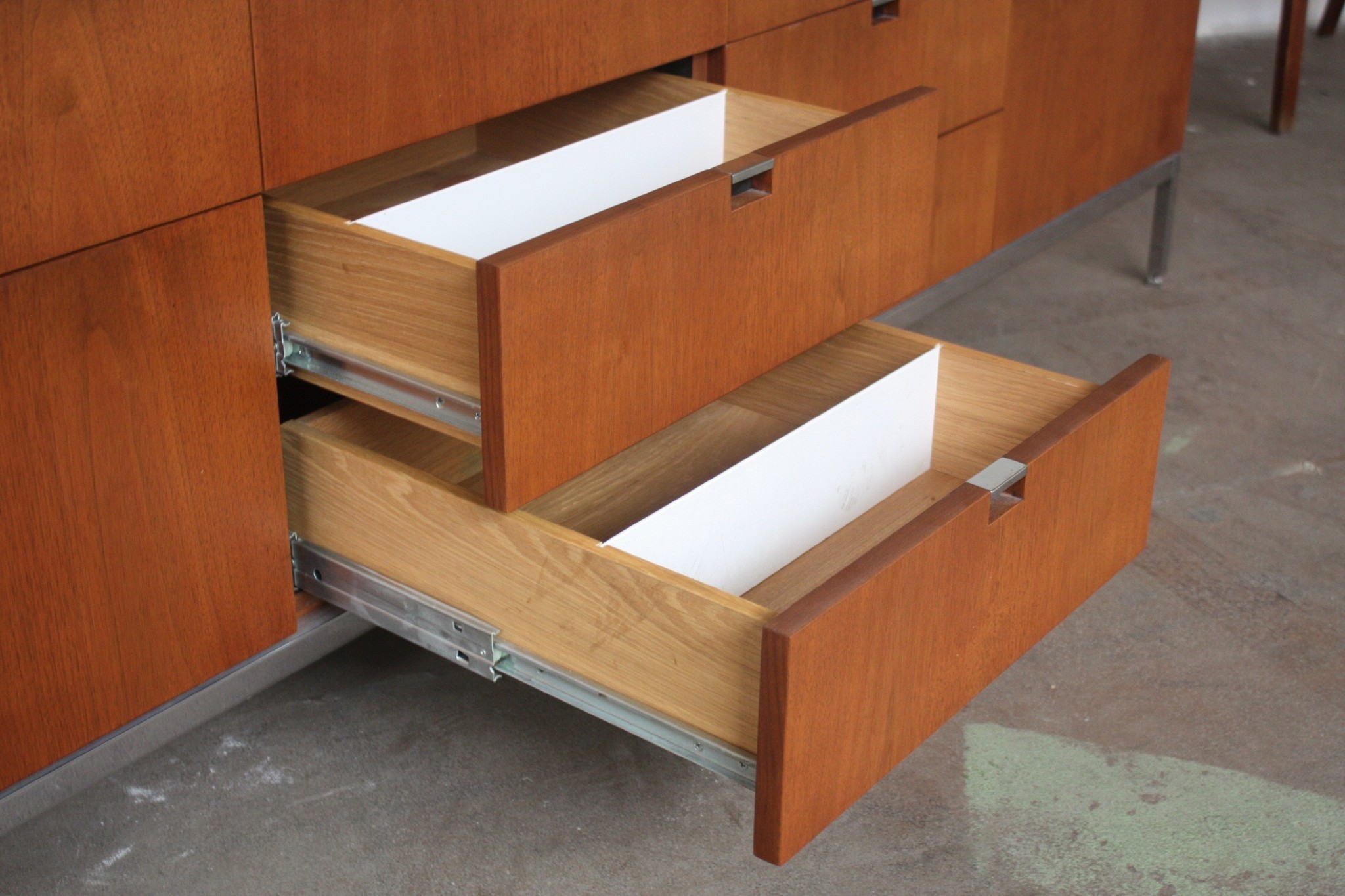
0, 0, 1200, 863
